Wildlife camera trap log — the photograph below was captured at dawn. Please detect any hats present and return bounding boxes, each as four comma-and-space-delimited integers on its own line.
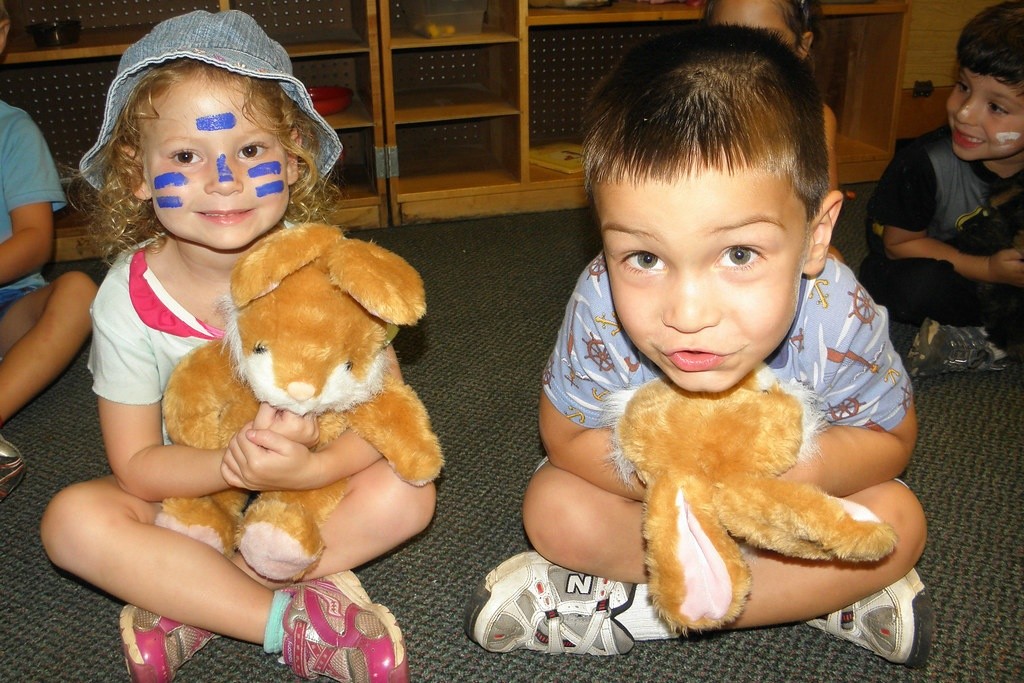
80, 11, 344, 190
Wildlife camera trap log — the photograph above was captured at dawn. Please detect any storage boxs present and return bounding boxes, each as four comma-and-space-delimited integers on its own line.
399, 0, 488, 40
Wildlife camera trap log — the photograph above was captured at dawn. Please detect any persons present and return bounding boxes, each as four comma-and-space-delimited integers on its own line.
464, 21, 931, 668
0, 3, 98, 501
701, 0, 843, 264
40, 11, 436, 683
859, 0, 1024, 379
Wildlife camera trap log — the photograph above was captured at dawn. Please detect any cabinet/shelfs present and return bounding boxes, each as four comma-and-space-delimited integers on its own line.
378, 0, 909, 228
0, 0, 388, 263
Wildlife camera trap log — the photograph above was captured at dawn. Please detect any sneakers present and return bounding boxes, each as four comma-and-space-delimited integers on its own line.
119, 604, 215, 683
804, 568, 934, 670
464, 549, 636, 656
900, 317, 1013, 378
278, 565, 409, 683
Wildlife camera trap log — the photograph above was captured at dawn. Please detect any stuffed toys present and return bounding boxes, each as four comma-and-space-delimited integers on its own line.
611, 361, 897, 633
155, 223, 445, 579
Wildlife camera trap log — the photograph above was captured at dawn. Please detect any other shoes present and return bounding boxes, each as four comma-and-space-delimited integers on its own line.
0, 437, 25, 501
